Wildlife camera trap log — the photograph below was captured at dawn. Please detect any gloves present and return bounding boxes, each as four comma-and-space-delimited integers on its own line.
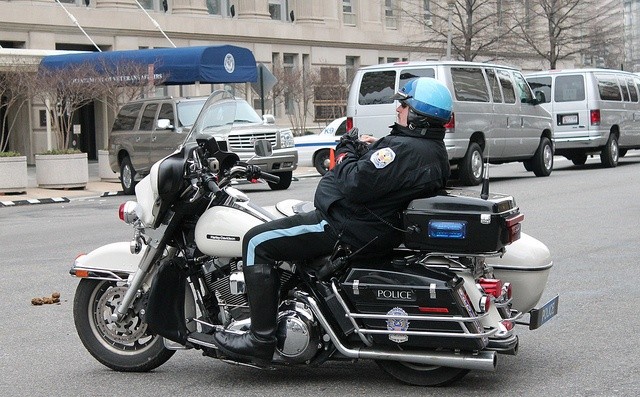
338, 127, 367, 156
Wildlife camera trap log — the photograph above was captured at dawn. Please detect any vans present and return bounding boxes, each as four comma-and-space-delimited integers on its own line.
524, 68, 640, 172
346, 61, 556, 186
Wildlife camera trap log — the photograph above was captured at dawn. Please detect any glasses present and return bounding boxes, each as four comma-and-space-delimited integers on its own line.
398, 99, 407, 107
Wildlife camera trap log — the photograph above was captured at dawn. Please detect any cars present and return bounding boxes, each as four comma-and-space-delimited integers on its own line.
293, 117, 347, 176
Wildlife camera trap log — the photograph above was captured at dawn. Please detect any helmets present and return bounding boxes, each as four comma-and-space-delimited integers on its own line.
392, 76, 453, 126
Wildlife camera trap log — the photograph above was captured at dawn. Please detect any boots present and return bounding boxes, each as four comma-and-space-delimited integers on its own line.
213, 264, 281, 366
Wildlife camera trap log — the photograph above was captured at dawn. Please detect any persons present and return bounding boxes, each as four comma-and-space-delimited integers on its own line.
213, 77, 453, 368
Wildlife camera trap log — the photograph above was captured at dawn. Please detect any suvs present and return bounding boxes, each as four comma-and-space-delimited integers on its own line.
108, 94, 298, 194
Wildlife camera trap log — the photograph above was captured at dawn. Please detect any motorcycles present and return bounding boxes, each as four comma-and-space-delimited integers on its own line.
69, 89, 558, 386
